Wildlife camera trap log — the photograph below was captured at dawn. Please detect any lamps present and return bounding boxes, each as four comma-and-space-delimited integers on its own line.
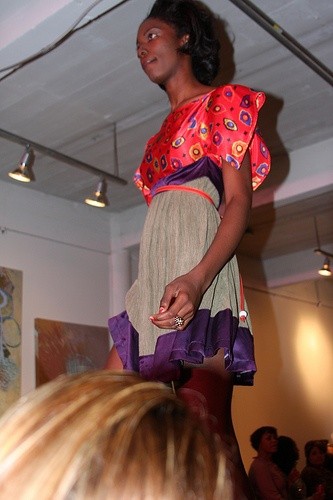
9, 143, 34, 182
84, 177, 109, 208
317, 259, 332, 277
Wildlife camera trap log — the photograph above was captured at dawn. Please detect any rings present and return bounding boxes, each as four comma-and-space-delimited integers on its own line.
174, 316, 184, 328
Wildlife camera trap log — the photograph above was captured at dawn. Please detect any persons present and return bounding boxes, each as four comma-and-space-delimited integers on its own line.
249, 426, 333, 499
105, 0, 270, 499
1, 369, 236, 499
